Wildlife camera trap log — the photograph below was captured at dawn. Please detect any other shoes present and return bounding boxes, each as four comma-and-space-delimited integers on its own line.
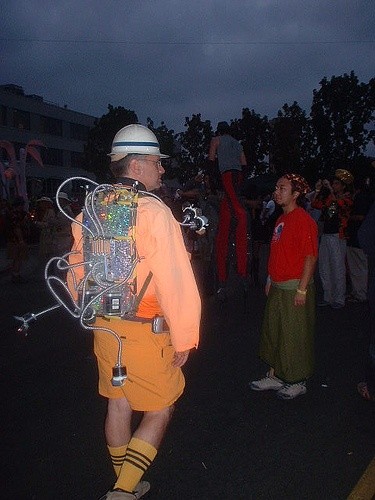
333, 303, 341, 308
98, 489, 137, 500
346, 297, 364, 304
317, 300, 328, 305
128, 480, 150, 497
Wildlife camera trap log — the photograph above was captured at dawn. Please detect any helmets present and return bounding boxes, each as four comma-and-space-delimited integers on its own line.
108, 124, 167, 159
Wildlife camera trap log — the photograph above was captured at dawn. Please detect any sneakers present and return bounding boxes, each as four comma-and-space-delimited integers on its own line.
251, 374, 283, 391
276, 382, 307, 399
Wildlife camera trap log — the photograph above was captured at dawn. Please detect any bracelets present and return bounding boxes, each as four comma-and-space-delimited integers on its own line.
19, 240, 24, 243
296, 289, 307, 295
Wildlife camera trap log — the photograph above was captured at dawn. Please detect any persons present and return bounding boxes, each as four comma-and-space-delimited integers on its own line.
356, 161, 375, 401
249, 174, 319, 399
210, 121, 249, 297
66, 125, 201, 500
0, 168, 368, 310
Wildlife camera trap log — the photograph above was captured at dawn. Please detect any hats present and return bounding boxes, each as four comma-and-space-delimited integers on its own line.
36, 196, 53, 203
57, 192, 72, 202
336, 168, 353, 184
